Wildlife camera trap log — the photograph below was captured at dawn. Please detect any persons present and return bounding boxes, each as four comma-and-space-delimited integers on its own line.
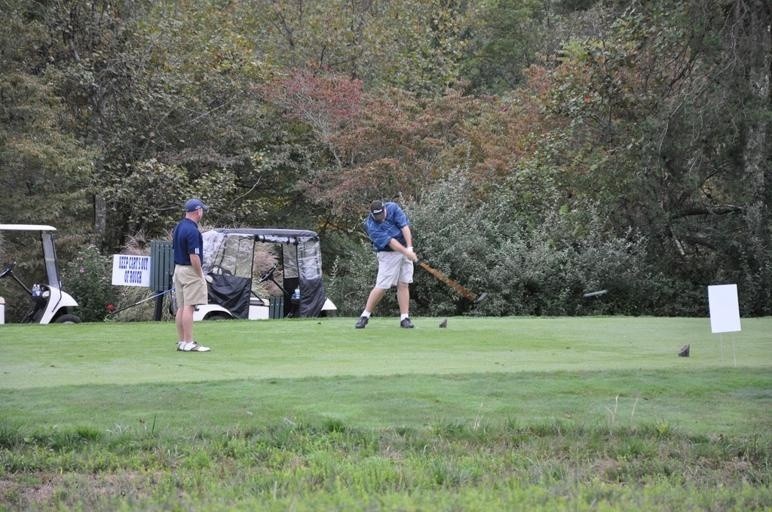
354, 198, 416, 330
172, 198, 211, 355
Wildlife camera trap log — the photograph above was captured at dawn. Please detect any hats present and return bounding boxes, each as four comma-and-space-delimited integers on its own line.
372, 201, 385, 220
182, 198, 209, 212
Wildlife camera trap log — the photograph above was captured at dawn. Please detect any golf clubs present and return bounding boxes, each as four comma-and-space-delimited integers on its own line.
103, 274, 213, 322
415, 258, 487, 303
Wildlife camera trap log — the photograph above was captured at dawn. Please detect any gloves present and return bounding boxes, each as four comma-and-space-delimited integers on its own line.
405, 247, 414, 264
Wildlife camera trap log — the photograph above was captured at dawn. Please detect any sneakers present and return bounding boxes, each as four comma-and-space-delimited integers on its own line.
401, 318, 414, 328
356, 316, 368, 328
183, 341, 210, 352
176, 341, 185, 351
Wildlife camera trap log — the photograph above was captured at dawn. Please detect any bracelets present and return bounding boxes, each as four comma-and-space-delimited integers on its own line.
408, 246, 413, 251
